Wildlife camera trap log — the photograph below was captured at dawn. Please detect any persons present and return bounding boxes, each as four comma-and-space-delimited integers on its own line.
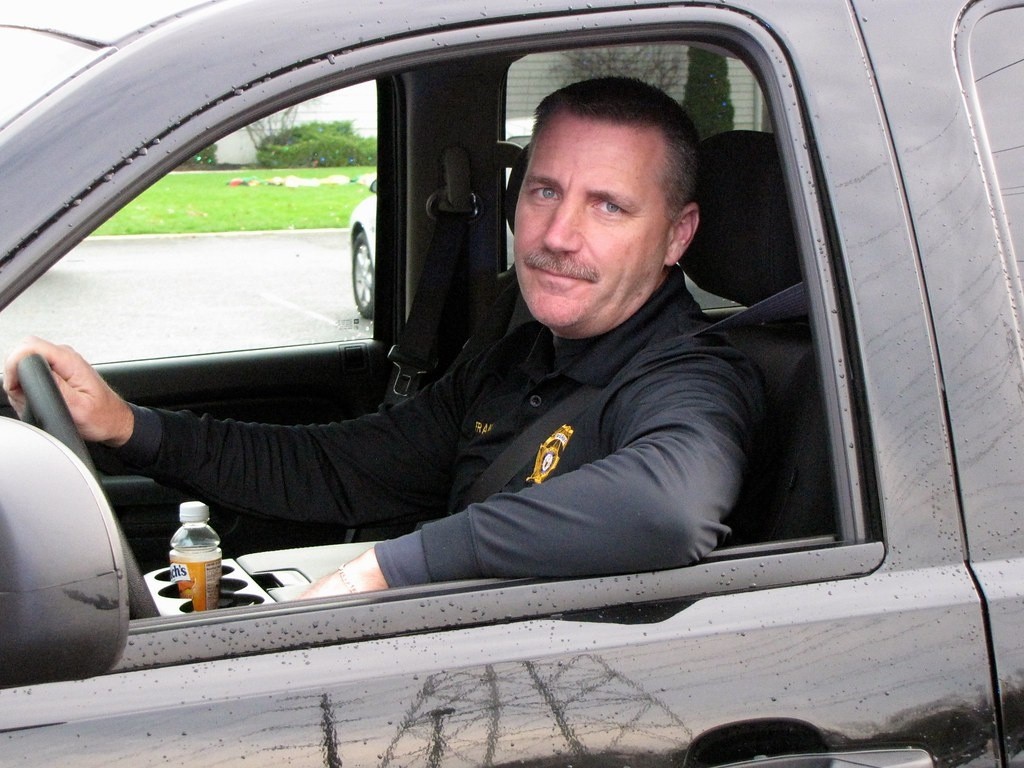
2, 77, 769, 599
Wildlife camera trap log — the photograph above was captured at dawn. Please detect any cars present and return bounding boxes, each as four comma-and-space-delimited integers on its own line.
347, 117, 536, 318
0, 0, 1024, 768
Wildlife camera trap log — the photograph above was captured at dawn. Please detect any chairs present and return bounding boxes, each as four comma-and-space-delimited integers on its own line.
679, 130, 844, 547
492, 141, 538, 343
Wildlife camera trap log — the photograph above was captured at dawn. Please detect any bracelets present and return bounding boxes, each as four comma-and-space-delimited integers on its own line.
338, 564, 359, 595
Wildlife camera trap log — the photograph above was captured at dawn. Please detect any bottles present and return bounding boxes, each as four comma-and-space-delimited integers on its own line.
169, 500, 222, 613
